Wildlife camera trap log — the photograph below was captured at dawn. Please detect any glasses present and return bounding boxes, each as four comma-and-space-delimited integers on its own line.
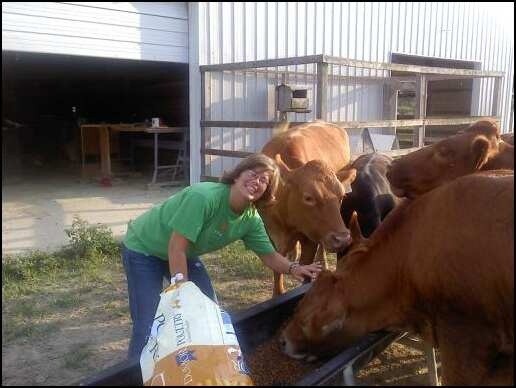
248, 169, 271, 187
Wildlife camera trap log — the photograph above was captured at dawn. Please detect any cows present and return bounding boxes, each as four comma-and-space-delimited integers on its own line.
387, 119, 515, 199
260, 122, 352, 298
279, 169, 515, 383
338, 150, 400, 261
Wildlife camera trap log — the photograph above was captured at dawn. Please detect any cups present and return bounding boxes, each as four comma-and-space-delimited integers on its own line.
152, 117, 160, 129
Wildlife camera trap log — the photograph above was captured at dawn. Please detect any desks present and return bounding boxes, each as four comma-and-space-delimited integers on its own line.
110, 124, 188, 184
80, 124, 110, 176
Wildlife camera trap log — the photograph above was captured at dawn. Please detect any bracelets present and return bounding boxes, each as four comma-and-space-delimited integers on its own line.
288, 262, 300, 273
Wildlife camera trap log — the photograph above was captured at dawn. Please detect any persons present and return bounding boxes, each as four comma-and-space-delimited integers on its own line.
118, 151, 323, 361
